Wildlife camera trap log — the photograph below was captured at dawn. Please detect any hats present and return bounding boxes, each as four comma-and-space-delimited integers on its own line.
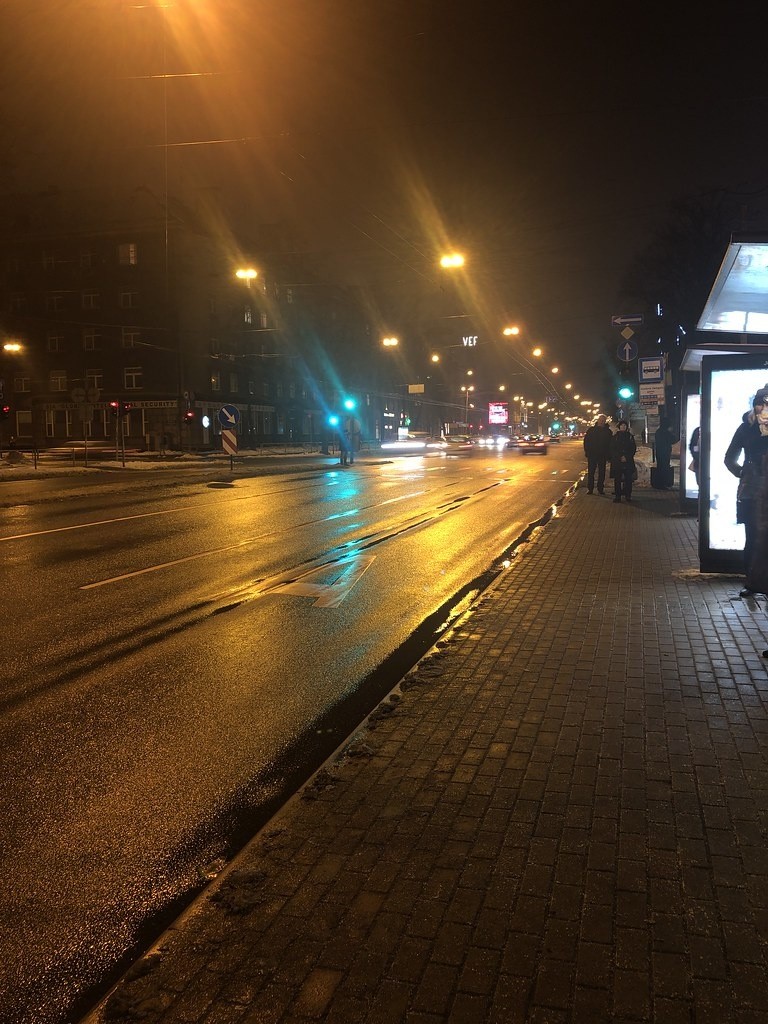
753, 383, 768, 405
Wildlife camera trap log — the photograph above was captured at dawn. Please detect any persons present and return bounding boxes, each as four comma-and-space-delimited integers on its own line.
340, 429, 351, 466
654, 417, 681, 470
584, 415, 613, 495
641, 429, 645, 442
689, 427, 699, 484
724, 388, 768, 597
612, 421, 636, 502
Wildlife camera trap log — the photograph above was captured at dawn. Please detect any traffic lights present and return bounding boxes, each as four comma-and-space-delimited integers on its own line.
619, 360, 638, 402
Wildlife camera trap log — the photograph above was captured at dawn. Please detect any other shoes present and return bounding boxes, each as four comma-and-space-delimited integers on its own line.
586, 490, 593, 494
762, 650, 768, 658
625, 496, 632, 501
613, 498, 621, 502
597, 490, 605, 494
740, 587, 754, 596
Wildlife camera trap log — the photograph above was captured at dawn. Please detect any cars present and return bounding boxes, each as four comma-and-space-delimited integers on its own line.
519, 433, 548, 455
426, 431, 520, 450
547, 433, 560, 443
